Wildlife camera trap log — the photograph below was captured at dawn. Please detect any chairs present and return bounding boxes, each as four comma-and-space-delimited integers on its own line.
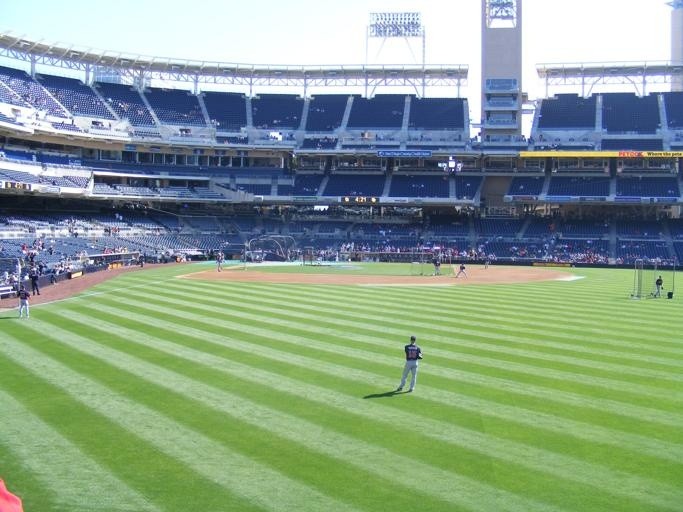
0, 68, 682, 280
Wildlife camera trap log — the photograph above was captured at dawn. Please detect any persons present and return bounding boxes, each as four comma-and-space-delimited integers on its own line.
1, 76, 681, 298
396, 334, 424, 394
16, 284, 31, 319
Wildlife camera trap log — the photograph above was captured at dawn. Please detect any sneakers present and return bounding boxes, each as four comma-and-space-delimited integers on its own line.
396, 388, 414, 392
